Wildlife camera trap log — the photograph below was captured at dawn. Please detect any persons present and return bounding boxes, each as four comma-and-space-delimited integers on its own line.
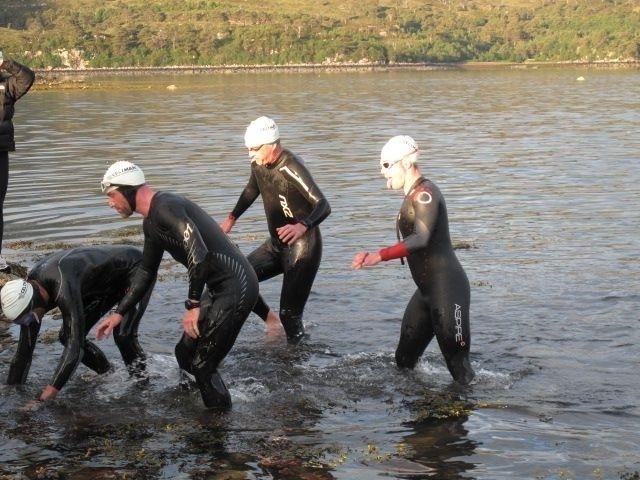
0, 51, 36, 254
96, 159, 261, 411
220, 116, 332, 346
0, 245, 158, 411
353, 134, 476, 385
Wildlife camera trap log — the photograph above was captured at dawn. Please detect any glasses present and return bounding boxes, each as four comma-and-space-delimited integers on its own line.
383, 160, 404, 169
101, 182, 131, 192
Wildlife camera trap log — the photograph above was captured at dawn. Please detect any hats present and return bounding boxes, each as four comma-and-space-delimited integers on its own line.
101, 161, 146, 194
0, 278, 34, 321
380, 134, 419, 165
244, 116, 280, 149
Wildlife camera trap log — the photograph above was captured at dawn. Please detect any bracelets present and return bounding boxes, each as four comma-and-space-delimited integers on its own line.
360, 251, 370, 265
